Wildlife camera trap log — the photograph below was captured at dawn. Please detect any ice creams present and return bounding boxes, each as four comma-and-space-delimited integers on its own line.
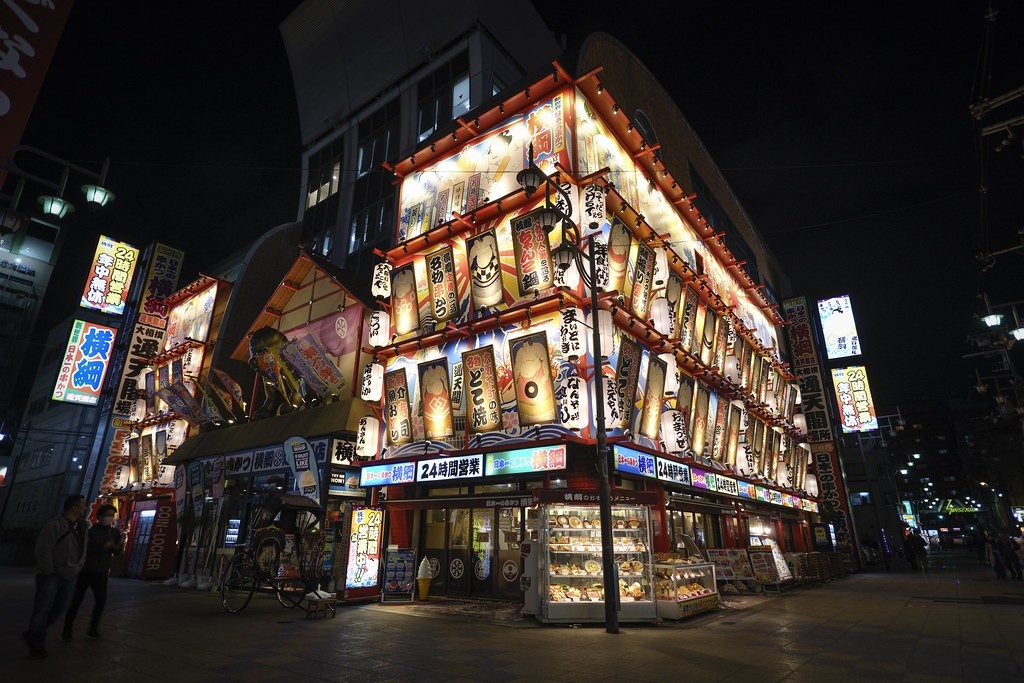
299, 470, 316, 499
416, 555, 432, 600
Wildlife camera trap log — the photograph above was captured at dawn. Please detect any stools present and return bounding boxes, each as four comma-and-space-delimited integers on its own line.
307, 600, 336, 620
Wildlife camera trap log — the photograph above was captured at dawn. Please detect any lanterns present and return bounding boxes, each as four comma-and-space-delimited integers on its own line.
111, 368, 153, 489
759, 357, 818, 497
554, 258, 579, 290
651, 248, 686, 454
555, 181, 580, 229
356, 415, 379, 459
371, 261, 393, 300
368, 309, 390, 348
580, 184, 621, 432
361, 362, 384, 402
558, 305, 587, 361
158, 348, 200, 485
722, 317, 753, 477
560, 374, 589, 432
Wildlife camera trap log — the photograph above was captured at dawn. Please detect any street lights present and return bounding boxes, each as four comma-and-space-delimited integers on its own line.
516, 141, 621, 635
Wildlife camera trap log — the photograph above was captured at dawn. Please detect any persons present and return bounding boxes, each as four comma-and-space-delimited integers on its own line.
20, 495, 123, 658
904, 530, 928, 572
860, 533, 870, 562
974, 528, 1024, 581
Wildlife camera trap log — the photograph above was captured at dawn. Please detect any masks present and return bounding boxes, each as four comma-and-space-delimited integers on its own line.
99, 516, 114, 526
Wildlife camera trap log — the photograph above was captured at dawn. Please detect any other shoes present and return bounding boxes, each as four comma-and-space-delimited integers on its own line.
59, 634, 73, 642
85, 629, 103, 640
22, 629, 48, 659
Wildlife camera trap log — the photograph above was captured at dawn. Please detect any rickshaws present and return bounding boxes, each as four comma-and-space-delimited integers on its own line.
220, 491, 336, 619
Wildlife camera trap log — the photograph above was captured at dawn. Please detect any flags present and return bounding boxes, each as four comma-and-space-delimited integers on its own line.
154, 334, 347, 428
174, 456, 227, 572
283, 435, 321, 529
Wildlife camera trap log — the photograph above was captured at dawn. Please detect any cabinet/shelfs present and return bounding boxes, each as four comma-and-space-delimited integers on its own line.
646, 561, 720, 623
538, 504, 656, 626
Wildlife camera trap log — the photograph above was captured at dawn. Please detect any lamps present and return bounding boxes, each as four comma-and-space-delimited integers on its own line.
381, 448, 387, 459
344, 436, 350, 446
389, 289, 541, 341
391, 71, 559, 176
423, 440, 431, 454
98, 481, 160, 498
167, 279, 210, 303
534, 424, 542, 440
476, 433, 483, 448
212, 392, 337, 427
400, 196, 492, 253
596, 84, 816, 500
154, 337, 192, 355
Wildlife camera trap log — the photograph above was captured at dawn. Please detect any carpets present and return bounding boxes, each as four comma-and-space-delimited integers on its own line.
415, 601, 528, 621
